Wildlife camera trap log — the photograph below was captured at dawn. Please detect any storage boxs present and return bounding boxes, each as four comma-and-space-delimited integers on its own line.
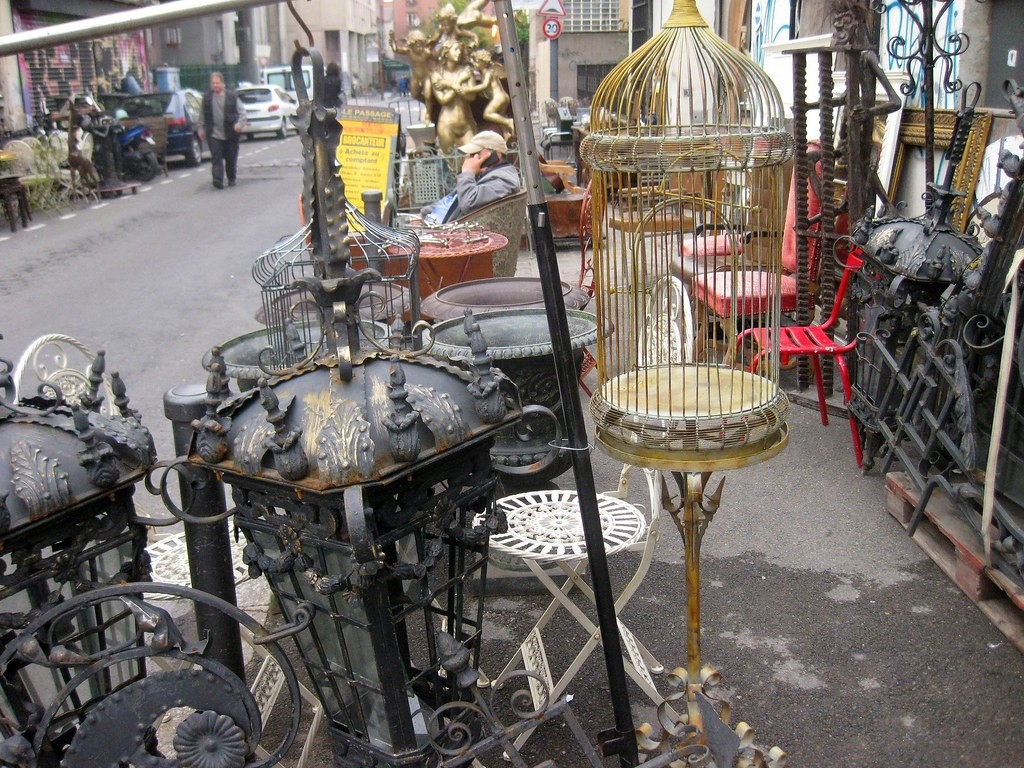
556, 107, 574, 140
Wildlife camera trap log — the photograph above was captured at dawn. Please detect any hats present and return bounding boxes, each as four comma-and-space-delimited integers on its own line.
457, 130, 508, 154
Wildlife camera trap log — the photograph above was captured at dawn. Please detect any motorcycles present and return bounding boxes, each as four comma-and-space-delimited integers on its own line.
61, 97, 159, 183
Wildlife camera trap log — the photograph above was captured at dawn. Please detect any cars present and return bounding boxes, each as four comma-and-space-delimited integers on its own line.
110, 86, 208, 166
233, 86, 300, 138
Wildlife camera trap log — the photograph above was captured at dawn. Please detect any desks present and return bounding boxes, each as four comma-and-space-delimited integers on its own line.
389, 230, 509, 289
0, 176, 32, 232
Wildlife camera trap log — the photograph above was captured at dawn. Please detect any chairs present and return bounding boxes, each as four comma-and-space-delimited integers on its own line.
669, 157, 794, 323
471, 274, 697, 760
691, 139, 823, 378
4, 128, 99, 220
610, 158, 703, 277
541, 130, 575, 160
450, 188, 528, 278
727, 244, 880, 469
679, 138, 782, 258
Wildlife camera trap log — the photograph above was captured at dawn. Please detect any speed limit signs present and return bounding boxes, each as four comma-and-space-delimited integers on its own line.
543, 18, 562, 40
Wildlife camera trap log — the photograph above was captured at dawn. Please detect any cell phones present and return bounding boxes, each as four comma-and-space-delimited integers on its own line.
480, 150, 499, 169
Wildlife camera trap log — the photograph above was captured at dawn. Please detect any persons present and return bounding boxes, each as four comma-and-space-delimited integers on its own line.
195, 71, 248, 189
389, 0, 517, 180
406, 130, 521, 228
322, 62, 343, 107
389, 75, 410, 97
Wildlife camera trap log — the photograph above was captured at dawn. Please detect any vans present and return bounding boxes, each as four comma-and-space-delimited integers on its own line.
260, 65, 327, 103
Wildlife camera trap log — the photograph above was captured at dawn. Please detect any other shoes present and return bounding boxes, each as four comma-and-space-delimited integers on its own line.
229, 179, 236, 186
213, 179, 223, 189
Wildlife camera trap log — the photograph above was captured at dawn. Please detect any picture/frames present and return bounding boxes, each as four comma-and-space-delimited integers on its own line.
890, 106, 994, 233
828, 91, 904, 216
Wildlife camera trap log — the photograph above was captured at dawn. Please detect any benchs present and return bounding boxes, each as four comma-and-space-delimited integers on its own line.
620, 173, 673, 210
